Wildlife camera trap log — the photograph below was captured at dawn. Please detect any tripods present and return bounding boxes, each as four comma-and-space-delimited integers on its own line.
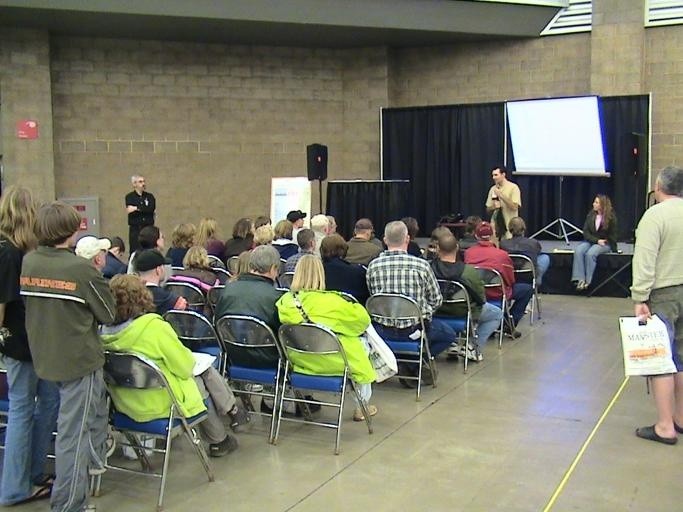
528, 180, 583, 246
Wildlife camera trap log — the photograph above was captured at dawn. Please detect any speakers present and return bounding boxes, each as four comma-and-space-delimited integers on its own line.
632, 131, 649, 177
307, 144, 328, 181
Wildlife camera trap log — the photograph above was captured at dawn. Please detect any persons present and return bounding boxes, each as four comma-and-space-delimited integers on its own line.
570, 192, 619, 295
0, 185, 60, 507
125, 174, 156, 261
484, 166, 522, 242
198, 210, 550, 388
629, 164, 683, 445
19, 199, 118, 511
76, 220, 398, 456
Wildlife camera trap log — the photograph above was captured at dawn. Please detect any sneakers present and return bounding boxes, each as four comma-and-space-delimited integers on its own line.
354, 405, 376, 420
259, 395, 323, 416
448, 343, 484, 361
210, 435, 236, 457
231, 408, 256, 431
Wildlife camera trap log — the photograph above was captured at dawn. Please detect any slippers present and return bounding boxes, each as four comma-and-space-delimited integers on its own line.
13, 474, 54, 505
636, 425, 678, 443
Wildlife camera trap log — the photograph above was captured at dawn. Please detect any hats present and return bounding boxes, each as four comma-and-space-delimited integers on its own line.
356, 218, 373, 229
75, 236, 112, 259
476, 221, 493, 236
287, 210, 306, 221
136, 249, 171, 271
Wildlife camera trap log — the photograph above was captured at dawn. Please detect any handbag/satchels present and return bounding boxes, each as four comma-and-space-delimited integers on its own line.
619, 313, 683, 377
368, 332, 398, 383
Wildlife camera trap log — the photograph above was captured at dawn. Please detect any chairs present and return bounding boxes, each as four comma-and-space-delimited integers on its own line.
162, 311, 249, 414
502, 254, 543, 325
472, 265, 516, 349
0, 328, 94, 495
95, 351, 215, 512
367, 294, 438, 402
216, 315, 305, 442
272, 323, 374, 456
436, 280, 480, 373
